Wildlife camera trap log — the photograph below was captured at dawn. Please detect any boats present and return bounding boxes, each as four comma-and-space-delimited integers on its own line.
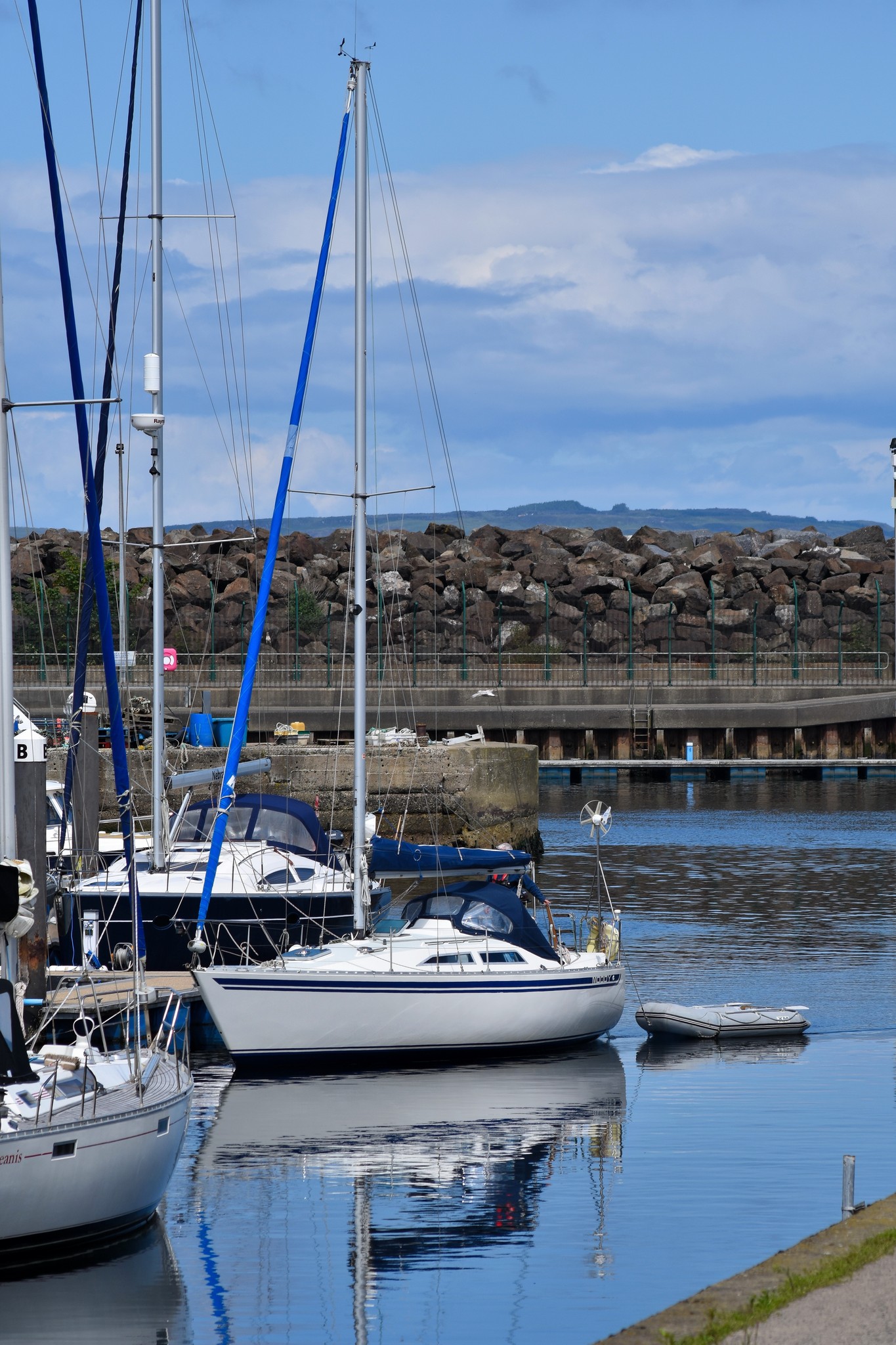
635, 1001, 813, 1042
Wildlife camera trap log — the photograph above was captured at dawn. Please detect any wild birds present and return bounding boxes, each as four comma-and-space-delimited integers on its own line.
465, 689, 499, 700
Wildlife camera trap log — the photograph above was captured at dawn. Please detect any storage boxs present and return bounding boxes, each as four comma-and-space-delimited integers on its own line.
274, 722, 314, 744
365, 733, 416, 746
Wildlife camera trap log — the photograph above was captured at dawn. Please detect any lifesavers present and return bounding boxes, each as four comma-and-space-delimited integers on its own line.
163, 653, 174, 668
586, 924, 619, 961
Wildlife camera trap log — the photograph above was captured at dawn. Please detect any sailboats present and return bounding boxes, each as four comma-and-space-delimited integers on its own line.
0, 0, 627, 1289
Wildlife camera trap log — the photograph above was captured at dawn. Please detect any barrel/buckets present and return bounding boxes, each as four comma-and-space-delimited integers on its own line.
108, 1002, 224, 1057
189, 713, 213, 747
291, 721, 305, 731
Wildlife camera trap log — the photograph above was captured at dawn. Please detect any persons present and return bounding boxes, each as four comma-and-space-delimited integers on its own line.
483, 843, 551, 932
